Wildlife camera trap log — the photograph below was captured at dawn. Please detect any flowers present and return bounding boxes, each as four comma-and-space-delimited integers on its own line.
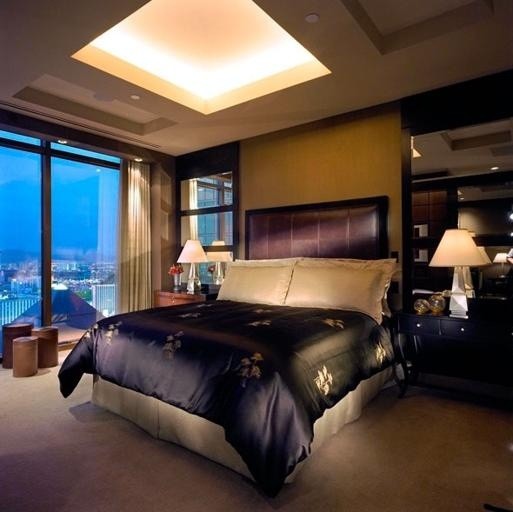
207, 265, 217, 281
168, 264, 184, 286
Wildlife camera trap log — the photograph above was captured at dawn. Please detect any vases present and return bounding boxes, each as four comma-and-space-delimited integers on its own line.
212, 272, 215, 280
174, 274, 181, 285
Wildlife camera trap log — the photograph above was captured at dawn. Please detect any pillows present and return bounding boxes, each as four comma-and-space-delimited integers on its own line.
284, 260, 395, 325
216, 257, 302, 306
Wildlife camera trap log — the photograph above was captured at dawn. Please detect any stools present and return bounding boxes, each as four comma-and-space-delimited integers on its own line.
2, 323, 33, 368
13, 336, 40, 377
32, 327, 58, 368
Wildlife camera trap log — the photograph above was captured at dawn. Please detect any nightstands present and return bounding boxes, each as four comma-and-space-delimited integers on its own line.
153, 284, 207, 308
395, 315, 489, 398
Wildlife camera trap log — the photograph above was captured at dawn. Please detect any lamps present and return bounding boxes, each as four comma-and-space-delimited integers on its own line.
490, 250, 513, 277
177, 240, 209, 295
462, 245, 492, 298
206, 241, 234, 285
427, 226, 489, 320
70, 0, 333, 118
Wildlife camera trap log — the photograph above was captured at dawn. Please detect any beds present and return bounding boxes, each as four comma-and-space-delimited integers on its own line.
58, 194, 409, 500
